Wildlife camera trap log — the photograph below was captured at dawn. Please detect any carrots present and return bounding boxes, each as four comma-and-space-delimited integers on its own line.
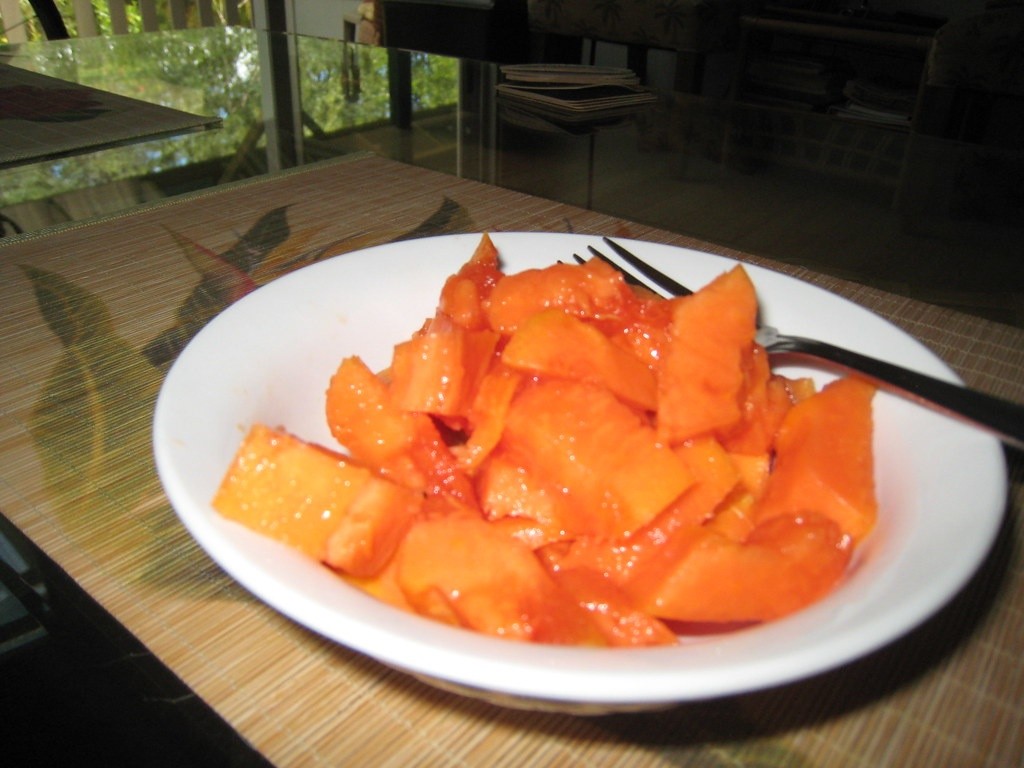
214, 233, 878, 645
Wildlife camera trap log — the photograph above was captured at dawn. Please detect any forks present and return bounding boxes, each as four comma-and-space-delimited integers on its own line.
556, 236, 1024, 453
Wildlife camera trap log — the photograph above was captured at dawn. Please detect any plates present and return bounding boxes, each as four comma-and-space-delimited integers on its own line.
151, 227, 1009, 720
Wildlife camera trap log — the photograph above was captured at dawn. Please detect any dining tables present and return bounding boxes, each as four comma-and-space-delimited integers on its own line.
0, 23, 1024, 768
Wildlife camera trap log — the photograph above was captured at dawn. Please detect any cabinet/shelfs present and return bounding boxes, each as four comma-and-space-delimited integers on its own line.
719, 13, 953, 213
